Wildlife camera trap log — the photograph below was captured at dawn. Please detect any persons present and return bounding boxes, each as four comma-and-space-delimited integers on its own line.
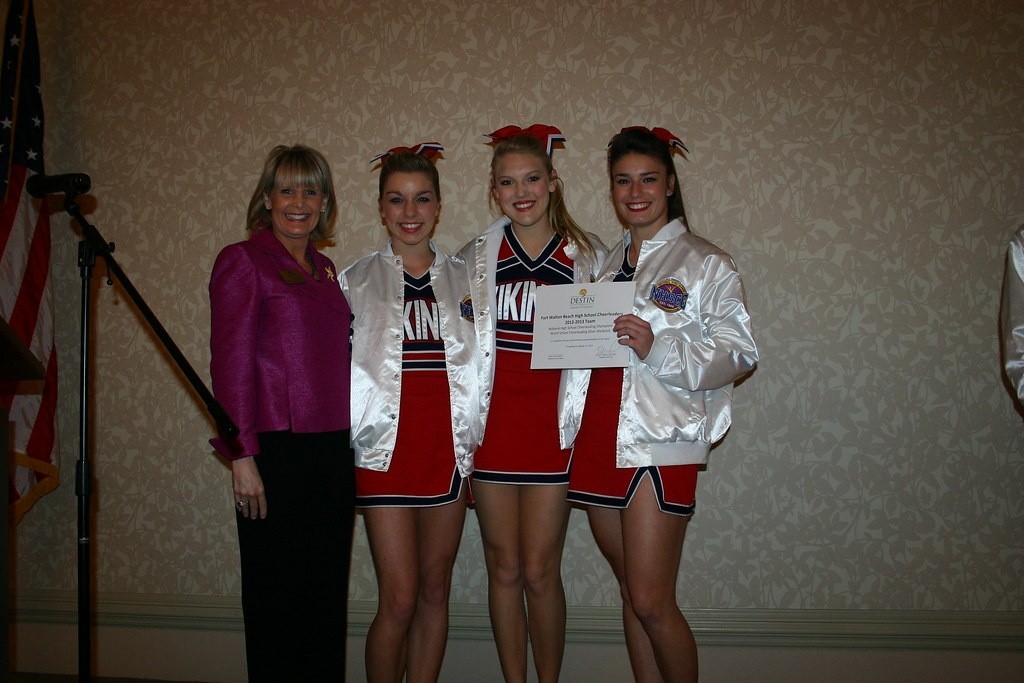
336, 141, 483, 683
452, 124, 611, 683
567, 126, 760, 683
207, 137, 355, 683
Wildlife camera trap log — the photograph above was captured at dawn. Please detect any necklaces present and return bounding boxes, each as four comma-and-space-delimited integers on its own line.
306, 252, 316, 277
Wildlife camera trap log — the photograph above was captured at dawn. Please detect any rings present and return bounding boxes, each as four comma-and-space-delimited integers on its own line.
237, 501, 249, 506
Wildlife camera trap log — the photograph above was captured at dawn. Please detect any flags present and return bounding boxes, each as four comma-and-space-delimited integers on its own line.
1, 1, 62, 525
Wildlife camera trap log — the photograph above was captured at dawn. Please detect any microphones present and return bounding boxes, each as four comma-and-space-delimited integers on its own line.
26, 173, 93, 197
